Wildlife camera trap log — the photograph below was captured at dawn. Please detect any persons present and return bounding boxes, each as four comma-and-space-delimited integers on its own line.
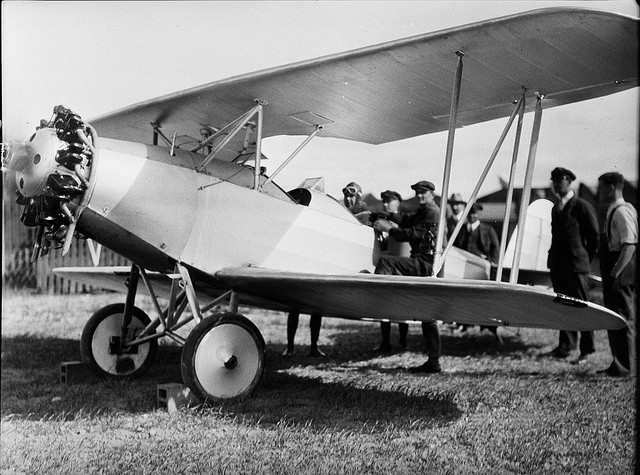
357, 181, 441, 374
597, 173, 637, 380
539, 165, 601, 366
456, 202, 499, 267
281, 312, 326, 359
447, 194, 467, 241
343, 183, 372, 227
372, 190, 409, 356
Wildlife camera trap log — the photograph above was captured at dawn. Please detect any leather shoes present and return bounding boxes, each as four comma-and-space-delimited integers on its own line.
373, 343, 392, 354
400, 346, 409, 352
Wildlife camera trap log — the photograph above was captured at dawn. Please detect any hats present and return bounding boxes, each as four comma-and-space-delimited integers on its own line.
411, 181, 435, 191
447, 193, 467, 207
469, 203, 483, 213
381, 190, 402, 203
550, 167, 576, 184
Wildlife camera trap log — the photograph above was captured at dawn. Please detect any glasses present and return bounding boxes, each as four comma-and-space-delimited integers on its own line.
342, 186, 362, 197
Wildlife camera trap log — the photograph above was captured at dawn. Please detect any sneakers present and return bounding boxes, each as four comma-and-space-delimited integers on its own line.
539, 347, 573, 362
309, 345, 326, 357
408, 360, 440, 373
282, 344, 294, 359
596, 368, 612, 377
570, 351, 596, 365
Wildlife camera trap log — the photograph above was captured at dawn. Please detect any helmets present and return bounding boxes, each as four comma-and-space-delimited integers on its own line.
342, 182, 363, 208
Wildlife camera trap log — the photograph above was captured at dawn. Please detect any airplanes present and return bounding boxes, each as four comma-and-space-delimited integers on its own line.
3, 7, 640, 407
